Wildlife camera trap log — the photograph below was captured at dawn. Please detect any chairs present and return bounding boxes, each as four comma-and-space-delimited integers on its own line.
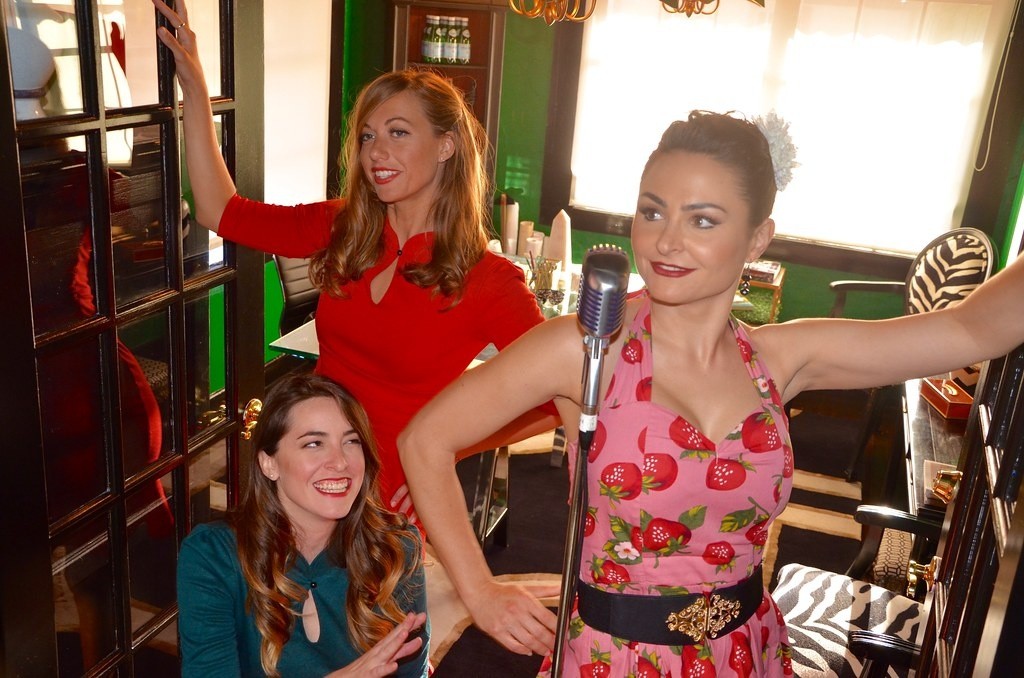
826, 227, 998, 484
769, 503, 945, 678
273, 255, 319, 339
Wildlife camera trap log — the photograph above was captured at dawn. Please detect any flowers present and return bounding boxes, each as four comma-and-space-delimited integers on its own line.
755, 110, 800, 191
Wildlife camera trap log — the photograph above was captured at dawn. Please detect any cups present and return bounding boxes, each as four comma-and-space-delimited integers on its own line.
526, 269, 551, 303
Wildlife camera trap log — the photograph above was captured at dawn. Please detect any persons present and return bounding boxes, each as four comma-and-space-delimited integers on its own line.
173, 369, 431, 678
397, 109, 1024, 678
152, 0, 563, 562
0, 27, 185, 678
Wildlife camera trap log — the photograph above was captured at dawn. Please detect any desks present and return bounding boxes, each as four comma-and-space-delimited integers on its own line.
267, 254, 644, 557
899, 373, 972, 595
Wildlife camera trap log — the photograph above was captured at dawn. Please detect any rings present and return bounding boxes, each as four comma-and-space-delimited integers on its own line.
175, 22, 186, 29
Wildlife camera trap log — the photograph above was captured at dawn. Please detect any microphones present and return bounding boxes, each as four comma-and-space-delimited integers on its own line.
576, 244, 631, 338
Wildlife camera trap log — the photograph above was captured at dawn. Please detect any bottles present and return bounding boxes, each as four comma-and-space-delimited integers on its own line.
429, 16, 441, 63
444, 17, 457, 64
441, 17, 448, 64
455, 17, 462, 64
459, 18, 471, 65
424, 16, 433, 63
420, 15, 429, 62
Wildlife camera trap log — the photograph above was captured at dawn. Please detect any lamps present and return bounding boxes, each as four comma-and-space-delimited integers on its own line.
6, 26, 55, 122
